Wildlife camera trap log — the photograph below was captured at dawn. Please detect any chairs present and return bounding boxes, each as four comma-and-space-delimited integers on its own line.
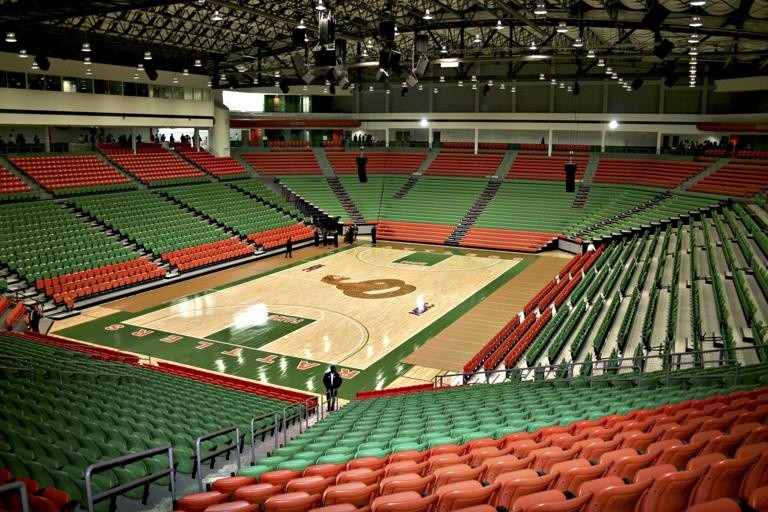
462, 189, 764, 378
0, 145, 318, 330
0, 329, 768, 511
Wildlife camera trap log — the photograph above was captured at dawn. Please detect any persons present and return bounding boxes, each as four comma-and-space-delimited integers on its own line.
15, 133, 20, 144
322, 365, 343, 413
341, 134, 351, 143
352, 221, 358, 242
19, 133, 25, 143
321, 228, 327, 246
365, 133, 372, 147
284, 237, 292, 258
262, 133, 268, 148
370, 223, 376, 244
331, 228, 338, 247
313, 228, 319, 246
353, 133, 357, 142
358, 133, 361, 146
24, 310, 31, 332
679, 138, 717, 156
361, 133, 366, 147
345, 224, 354, 245
77, 133, 202, 149
34, 133, 40, 143
7, 133, 16, 144
540, 136, 544, 145
29, 304, 41, 334
